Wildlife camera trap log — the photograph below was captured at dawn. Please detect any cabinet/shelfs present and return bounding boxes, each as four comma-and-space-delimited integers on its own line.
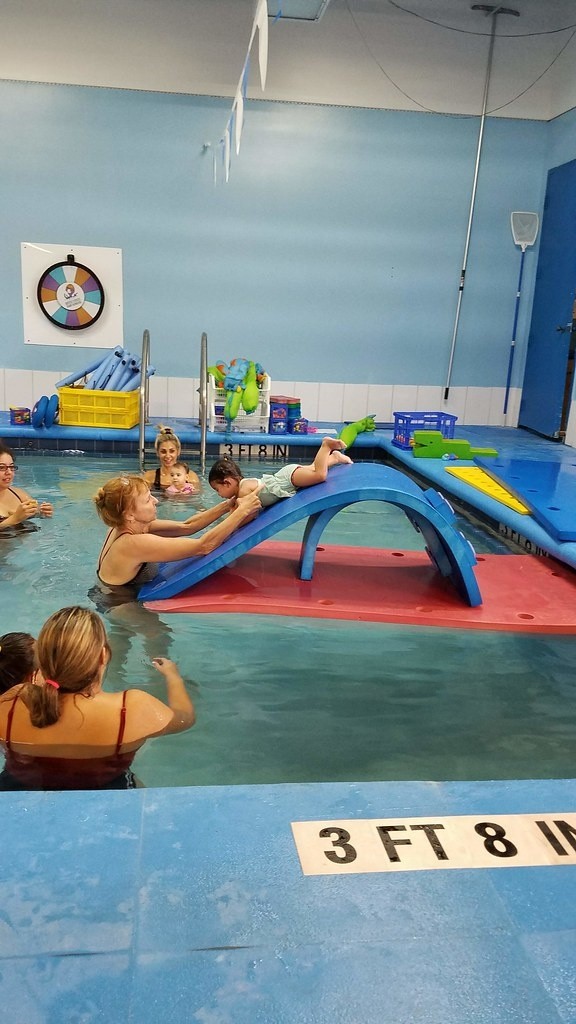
206, 371, 271, 434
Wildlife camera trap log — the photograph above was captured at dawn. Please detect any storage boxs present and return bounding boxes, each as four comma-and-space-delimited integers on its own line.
268, 395, 309, 435
56, 387, 140, 430
394, 411, 459, 449
9, 408, 30, 426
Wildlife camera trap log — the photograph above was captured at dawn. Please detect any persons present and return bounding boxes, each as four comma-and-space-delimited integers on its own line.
0, 448, 53, 531
209, 437, 353, 535
140, 424, 200, 496
88, 475, 266, 602
0, 608, 195, 792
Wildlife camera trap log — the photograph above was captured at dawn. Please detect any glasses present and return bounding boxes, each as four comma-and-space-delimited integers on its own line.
118, 472, 130, 513
0, 465, 18, 471
